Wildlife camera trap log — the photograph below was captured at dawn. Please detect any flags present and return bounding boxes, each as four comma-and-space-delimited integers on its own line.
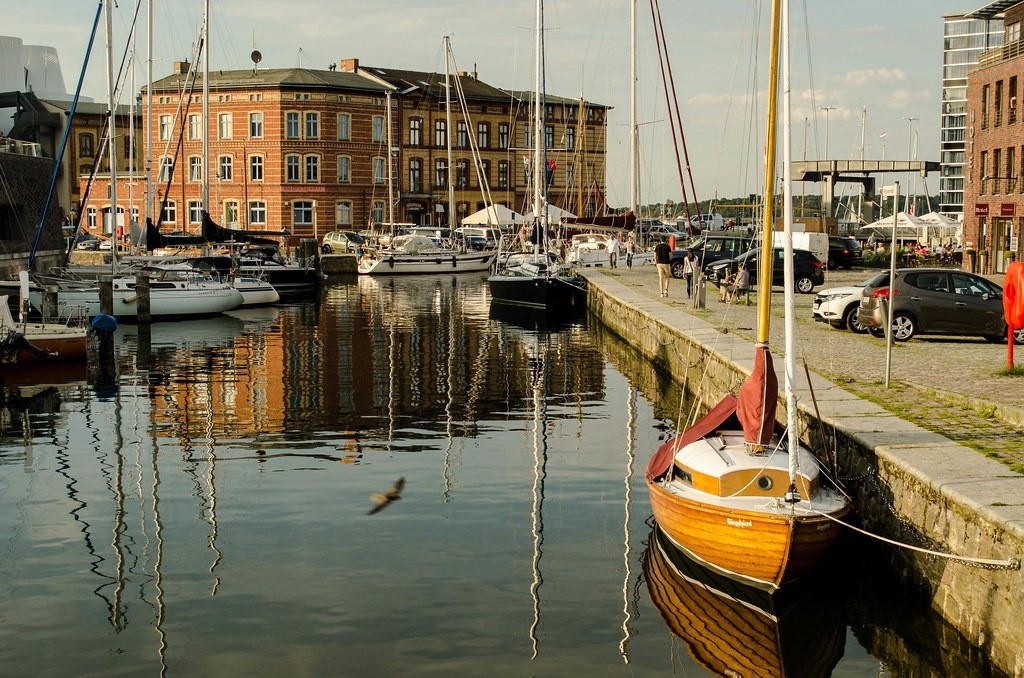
549, 161, 556, 170
880, 131, 886, 142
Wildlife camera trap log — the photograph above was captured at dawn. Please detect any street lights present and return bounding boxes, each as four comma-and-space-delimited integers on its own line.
818, 107, 836, 160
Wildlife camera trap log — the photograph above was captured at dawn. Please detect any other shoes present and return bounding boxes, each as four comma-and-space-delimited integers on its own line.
660, 293, 663, 297
719, 299, 725, 301
665, 291, 668, 297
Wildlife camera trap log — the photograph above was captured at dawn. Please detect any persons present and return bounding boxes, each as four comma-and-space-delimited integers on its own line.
280, 225, 290, 249
619, 235, 635, 270
654, 236, 672, 298
848, 231, 963, 265
605, 234, 621, 268
720, 262, 749, 304
683, 248, 699, 300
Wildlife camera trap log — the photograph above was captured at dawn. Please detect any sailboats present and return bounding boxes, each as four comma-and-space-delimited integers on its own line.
644, 1, 852, 594
356, 36, 507, 272
488, 299, 587, 658
498, 0, 656, 268
486, 1, 586, 311
644, 519, 848, 678
0, 0, 328, 363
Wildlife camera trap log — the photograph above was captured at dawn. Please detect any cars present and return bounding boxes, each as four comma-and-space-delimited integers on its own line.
856, 268, 1024, 344
647, 226, 688, 242
62, 226, 112, 250
827, 236, 865, 270
321, 231, 365, 254
811, 269, 989, 335
633, 219, 664, 232
704, 247, 825, 294
670, 236, 762, 279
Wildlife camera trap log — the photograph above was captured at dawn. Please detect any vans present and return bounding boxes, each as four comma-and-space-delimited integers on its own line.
685, 214, 724, 230
456, 227, 502, 247
746, 231, 829, 266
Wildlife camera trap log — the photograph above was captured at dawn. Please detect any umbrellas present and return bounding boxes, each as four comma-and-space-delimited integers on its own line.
917, 212, 961, 237
861, 212, 938, 249
462, 204, 525, 227
525, 204, 578, 224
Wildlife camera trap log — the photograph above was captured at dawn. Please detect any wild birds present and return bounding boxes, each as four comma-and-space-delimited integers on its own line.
368, 475, 405, 516
